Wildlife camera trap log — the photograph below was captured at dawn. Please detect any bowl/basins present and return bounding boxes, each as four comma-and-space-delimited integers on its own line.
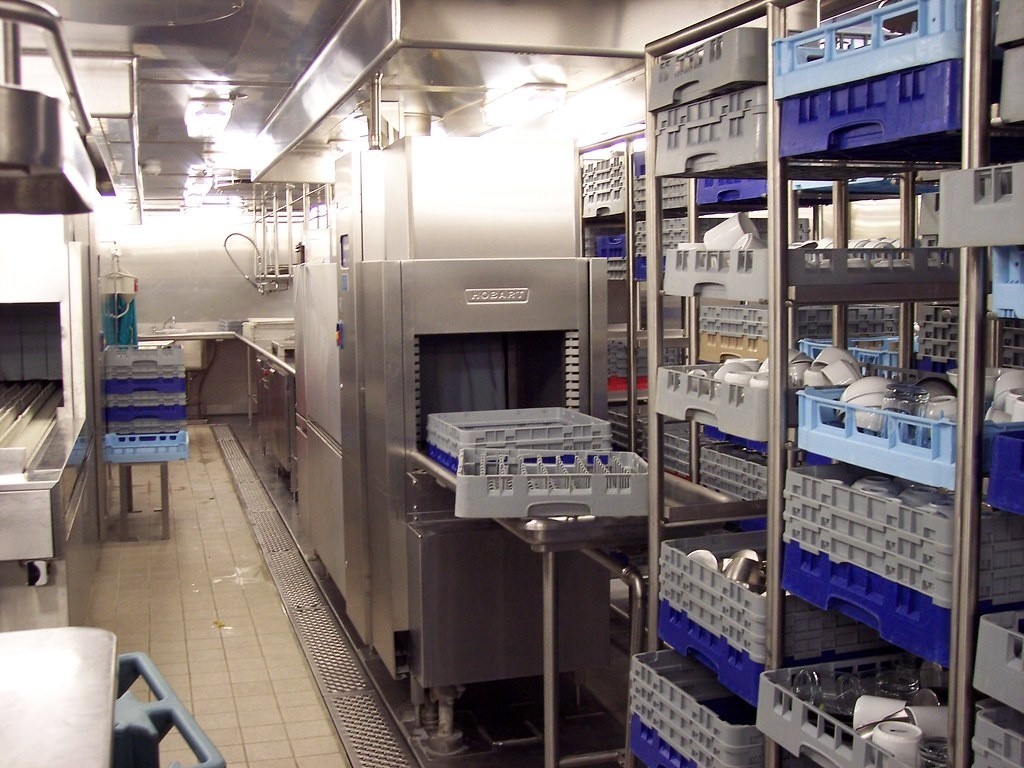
840, 368, 1023, 438
688, 549, 794, 598
688, 347, 862, 399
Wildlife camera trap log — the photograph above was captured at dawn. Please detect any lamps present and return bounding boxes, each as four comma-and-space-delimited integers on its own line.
185, 98, 234, 138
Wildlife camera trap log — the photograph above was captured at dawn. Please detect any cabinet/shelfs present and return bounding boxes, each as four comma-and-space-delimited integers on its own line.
580, 127, 916, 485
636, 0, 1001, 768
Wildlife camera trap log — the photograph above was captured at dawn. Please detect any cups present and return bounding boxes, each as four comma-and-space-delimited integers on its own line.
792, 662, 950, 768
677, 213, 910, 268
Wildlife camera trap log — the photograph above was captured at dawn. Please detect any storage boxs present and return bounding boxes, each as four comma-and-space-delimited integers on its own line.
103, 342, 190, 464
585, 0, 1024, 768
427, 400, 652, 518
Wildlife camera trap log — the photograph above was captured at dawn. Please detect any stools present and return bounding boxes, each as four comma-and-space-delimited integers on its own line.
117, 460, 168, 543
